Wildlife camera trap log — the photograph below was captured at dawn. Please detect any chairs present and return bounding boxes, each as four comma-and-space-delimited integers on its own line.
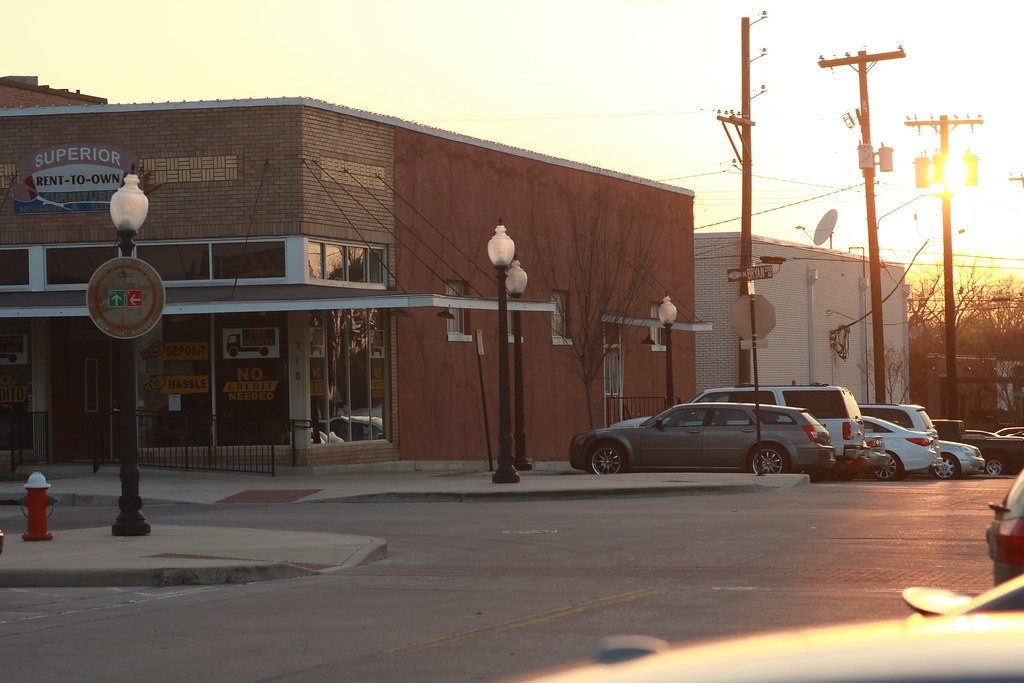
751, 413, 775, 424
719, 411, 729, 425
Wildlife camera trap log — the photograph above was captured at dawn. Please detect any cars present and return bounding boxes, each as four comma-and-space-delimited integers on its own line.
929, 417, 1023, 594
861, 414, 938, 479
836, 431, 889, 479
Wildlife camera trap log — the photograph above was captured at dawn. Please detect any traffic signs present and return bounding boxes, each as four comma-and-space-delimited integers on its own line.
727, 264, 774, 285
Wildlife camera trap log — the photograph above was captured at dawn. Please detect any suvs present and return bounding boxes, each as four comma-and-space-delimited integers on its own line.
615, 380, 864, 474
569, 403, 836, 475
857, 402, 939, 458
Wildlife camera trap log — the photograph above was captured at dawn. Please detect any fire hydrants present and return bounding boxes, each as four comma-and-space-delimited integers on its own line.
18, 468, 58, 541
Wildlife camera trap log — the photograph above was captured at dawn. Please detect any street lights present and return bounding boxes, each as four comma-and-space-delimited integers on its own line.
108, 162, 152, 536
488, 215, 521, 485
503, 255, 535, 469
657, 291, 678, 409
